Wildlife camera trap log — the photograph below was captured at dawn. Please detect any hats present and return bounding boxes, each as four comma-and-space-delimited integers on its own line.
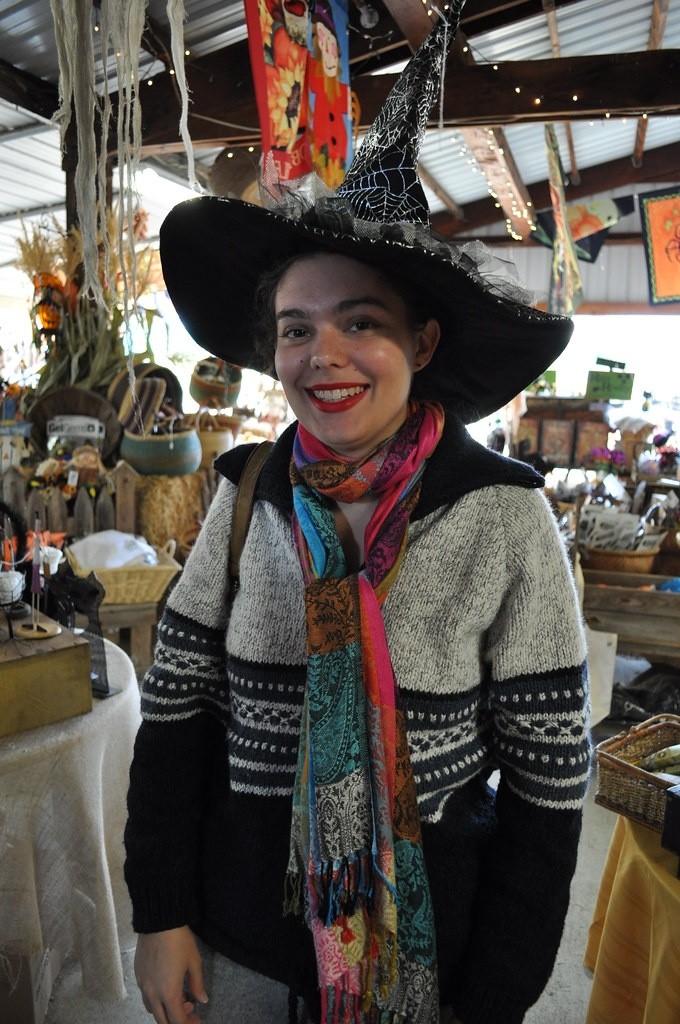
159, 0, 574, 426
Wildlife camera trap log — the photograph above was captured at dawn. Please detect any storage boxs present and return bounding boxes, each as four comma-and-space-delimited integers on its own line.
0, 618, 93, 737
583, 569, 680, 659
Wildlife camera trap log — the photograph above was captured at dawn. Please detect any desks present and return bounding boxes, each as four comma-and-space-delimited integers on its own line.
76, 604, 157, 683
19, 464, 206, 550
0, 627, 143, 1024
582, 814, 680, 1024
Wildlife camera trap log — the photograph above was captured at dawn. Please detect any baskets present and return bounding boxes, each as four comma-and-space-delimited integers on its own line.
111, 355, 242, 474
64, 537, 181, 605
580, 544, 661, 573
594, 714, 679, 832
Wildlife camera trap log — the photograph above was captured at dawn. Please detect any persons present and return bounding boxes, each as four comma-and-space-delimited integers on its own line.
123, 198, 591, 1024
